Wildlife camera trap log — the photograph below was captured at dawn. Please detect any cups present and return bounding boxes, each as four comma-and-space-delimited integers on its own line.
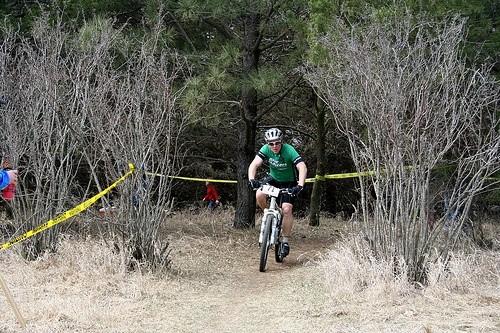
2, 167, 14, 199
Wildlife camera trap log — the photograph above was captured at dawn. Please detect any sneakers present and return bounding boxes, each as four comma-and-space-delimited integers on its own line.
281, 242, 290, 255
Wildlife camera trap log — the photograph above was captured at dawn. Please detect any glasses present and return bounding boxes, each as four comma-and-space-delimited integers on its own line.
268, 142, 281, 146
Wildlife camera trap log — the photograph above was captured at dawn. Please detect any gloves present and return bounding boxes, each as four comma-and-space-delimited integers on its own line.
288, 185, 303, 197
250, 179, 263, 191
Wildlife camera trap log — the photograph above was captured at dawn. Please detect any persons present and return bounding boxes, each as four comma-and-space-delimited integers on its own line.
247, 128, 307, 256
202, 176, 219, 212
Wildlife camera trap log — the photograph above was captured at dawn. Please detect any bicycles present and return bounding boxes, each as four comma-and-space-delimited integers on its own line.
247, 181, 295, 272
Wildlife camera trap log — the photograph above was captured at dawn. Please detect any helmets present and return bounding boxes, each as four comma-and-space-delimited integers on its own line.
264, 128, 283, 142
